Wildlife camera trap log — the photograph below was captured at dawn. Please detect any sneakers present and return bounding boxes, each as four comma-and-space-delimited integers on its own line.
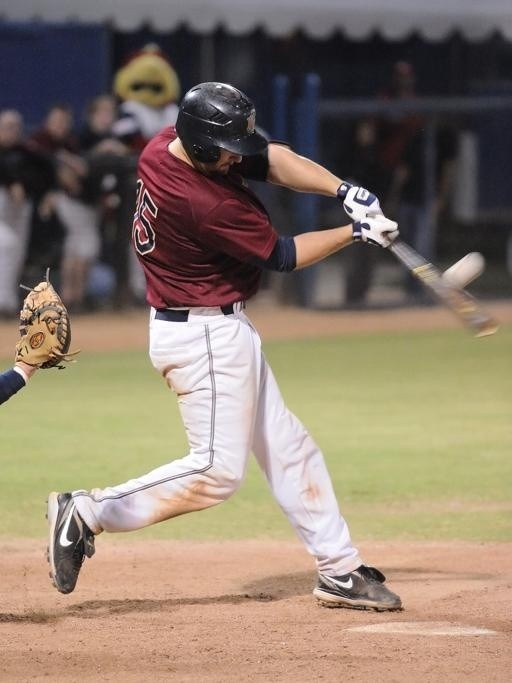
47, 490, 95, 594
313, 565, 401, 608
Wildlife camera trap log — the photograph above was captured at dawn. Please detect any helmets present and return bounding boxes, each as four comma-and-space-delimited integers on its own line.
175, 81, 267, 163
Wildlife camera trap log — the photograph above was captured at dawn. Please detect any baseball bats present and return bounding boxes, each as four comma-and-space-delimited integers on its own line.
385, 231, 500, 337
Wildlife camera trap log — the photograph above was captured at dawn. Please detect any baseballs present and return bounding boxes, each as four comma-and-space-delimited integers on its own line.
443, 251, 485, 287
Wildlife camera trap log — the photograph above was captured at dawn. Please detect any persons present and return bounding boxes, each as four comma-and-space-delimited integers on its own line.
41, 76, 407, 615
2, 57, 182, 311
341, 59, 458, 309
0, 266, 82, 404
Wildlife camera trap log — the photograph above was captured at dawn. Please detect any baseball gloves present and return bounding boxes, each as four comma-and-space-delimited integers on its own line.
15, 267, 80, 369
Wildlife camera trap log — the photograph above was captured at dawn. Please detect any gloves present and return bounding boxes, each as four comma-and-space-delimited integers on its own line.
351, 213, 398, 249
338, 182, 383, 222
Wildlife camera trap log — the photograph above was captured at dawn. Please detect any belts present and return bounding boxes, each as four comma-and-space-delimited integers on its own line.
154, 303, 233, 320
